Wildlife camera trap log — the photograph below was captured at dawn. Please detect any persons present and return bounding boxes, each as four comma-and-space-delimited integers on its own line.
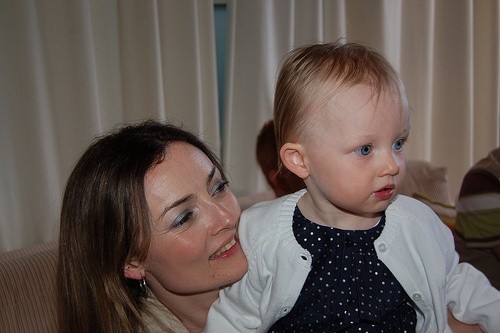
203, 44, 500, 333
447, 145, 500, 333
59, 119, 248, 333
